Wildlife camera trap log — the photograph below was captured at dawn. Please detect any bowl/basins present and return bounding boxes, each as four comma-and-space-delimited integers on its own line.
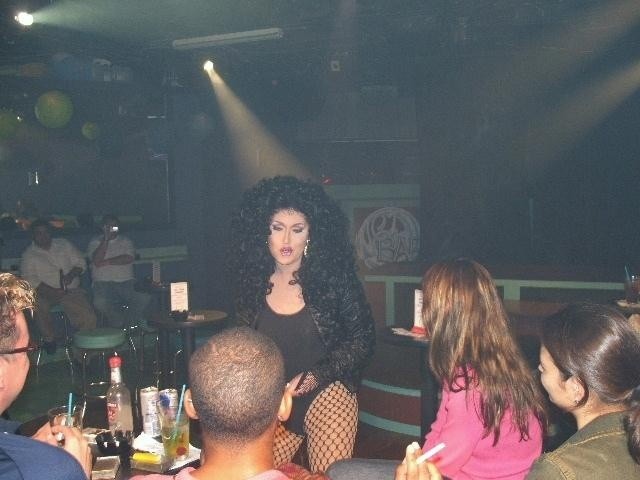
96, 429, 134, 456
168, 310, 190, 323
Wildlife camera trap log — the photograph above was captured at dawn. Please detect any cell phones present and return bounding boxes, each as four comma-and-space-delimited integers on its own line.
109, 226, 119, 233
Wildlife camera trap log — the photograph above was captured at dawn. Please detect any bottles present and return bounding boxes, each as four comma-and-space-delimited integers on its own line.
105, 358, 133, 434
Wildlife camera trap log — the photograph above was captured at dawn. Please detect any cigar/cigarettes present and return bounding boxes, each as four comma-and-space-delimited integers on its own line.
416, 441, 447, 465
56, 415, 67, 442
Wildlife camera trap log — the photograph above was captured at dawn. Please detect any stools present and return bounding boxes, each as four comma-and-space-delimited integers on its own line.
14, 278, 192, 402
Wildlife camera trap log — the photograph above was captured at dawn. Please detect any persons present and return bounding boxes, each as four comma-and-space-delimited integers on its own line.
85, 213, 137, 329
394, 441, 443, 480
18, 219, 97, 355
133, 325, 293, 480
419, 257, 547, 480
221, 174, 378, 475
520, 300, 640, 480
0, 268, 96, 479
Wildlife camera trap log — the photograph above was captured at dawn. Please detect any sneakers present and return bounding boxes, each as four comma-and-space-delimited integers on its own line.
47, 341, 56, 355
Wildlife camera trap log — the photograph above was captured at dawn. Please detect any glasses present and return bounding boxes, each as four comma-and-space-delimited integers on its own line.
1, 343, 42, 367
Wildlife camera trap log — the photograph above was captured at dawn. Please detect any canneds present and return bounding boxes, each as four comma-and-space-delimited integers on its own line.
140, 386, 162, 437
160, 388, 178, 425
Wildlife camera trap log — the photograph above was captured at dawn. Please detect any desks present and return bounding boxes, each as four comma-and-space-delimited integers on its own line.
148, 307, 226, 374
379, 320, 450, 454
13, 401, 211, 480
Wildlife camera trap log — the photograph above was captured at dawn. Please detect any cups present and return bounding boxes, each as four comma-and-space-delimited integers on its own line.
157, 397, 190, 463
623, 275, 640, 304
46, 405, 84, 447
